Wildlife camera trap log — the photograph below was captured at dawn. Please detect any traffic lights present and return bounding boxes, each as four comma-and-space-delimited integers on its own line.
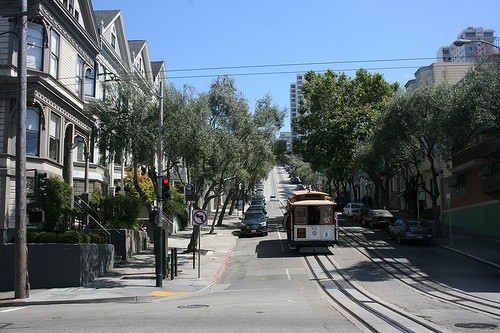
162, 177, 171, 201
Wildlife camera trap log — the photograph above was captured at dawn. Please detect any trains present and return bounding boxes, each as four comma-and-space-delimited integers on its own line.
282, 187, 338, 253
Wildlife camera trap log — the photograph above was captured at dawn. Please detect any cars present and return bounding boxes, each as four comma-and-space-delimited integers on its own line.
361, 210, 394, 228
353, 207, 372, 224
386, 218, 433, 244
239, 181, 268, 236
283, 162, 299, 183
342, 203, 365, 219
298, 185, 304, 190
270, 195, 277, 201
